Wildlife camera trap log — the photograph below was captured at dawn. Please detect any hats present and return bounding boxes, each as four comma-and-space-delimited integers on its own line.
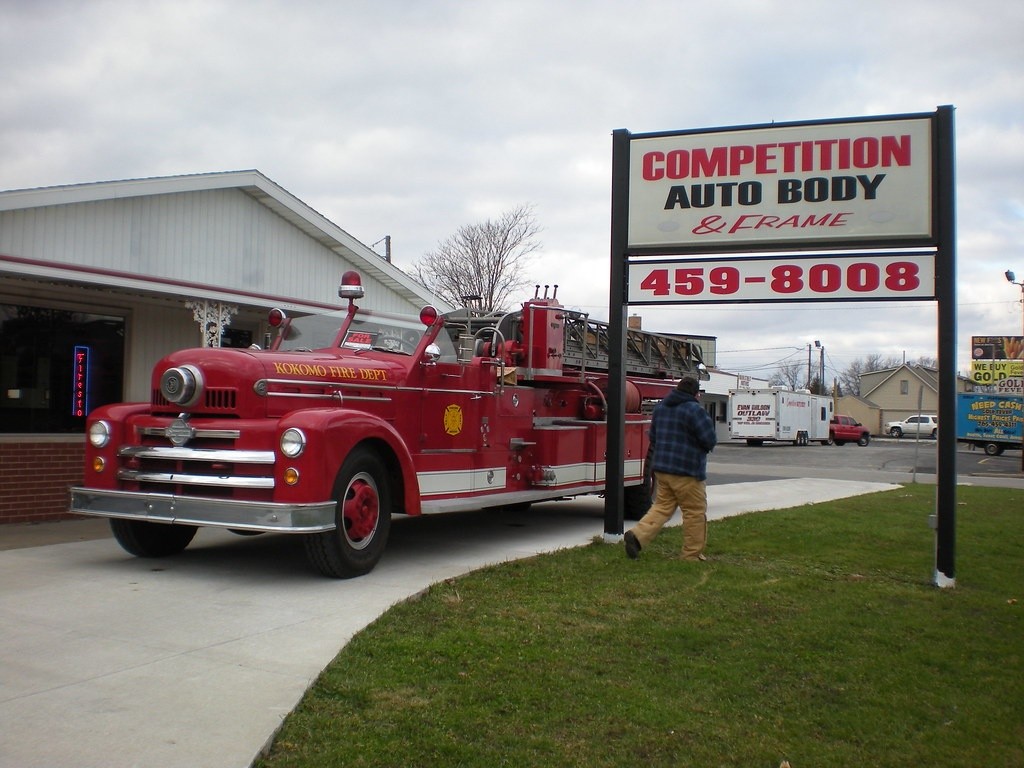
677, 377, 699, 396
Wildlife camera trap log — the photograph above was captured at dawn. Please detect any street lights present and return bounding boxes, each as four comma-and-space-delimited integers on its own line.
1005, 271, 1023, 337
815, 340, 824, 394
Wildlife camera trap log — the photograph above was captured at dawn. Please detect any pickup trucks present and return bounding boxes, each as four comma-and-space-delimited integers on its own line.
821, 415, 871, 447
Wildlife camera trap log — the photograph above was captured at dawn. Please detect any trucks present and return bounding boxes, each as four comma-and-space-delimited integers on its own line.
955, 392, 1023, 456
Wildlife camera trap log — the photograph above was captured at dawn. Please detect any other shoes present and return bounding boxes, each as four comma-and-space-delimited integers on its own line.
624, 530, 641, 560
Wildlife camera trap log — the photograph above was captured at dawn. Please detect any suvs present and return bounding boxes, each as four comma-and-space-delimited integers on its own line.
885, 414, 937, 440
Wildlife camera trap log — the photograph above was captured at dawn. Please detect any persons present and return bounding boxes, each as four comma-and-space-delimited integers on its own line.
623, 377, 718, 562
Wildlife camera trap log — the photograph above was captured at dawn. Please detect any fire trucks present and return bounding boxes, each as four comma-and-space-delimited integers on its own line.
66, 268, 710, 580
